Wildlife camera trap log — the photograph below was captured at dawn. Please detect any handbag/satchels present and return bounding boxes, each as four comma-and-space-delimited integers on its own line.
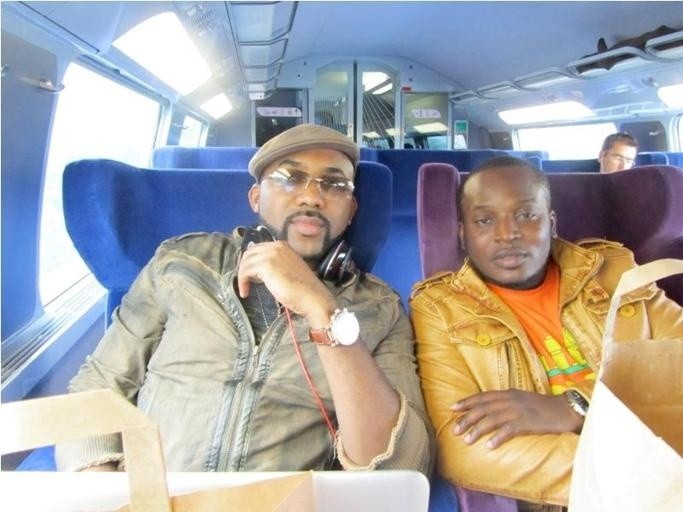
0, 387, 430, 512
567, 258, 683, 512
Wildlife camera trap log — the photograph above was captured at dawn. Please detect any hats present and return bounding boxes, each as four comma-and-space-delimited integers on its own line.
246, 122, 361, 181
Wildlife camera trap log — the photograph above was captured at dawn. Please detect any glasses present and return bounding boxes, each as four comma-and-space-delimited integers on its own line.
259, 166, 354, 204
614, 155, 636, 167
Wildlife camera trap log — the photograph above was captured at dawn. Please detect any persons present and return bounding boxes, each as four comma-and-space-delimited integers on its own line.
595, 132, 639, 174
406, 155, 682, 510
49, 122, 437, 489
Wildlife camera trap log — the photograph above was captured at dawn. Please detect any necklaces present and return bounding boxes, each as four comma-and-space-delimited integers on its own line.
253, 283, 281, 330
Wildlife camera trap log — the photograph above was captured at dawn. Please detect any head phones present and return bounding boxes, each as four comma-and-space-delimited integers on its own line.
240, 224, 358, 289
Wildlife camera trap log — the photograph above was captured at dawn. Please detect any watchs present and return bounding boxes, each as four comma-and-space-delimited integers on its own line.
306, 308, 360, 348
566, 390, 588, 416
